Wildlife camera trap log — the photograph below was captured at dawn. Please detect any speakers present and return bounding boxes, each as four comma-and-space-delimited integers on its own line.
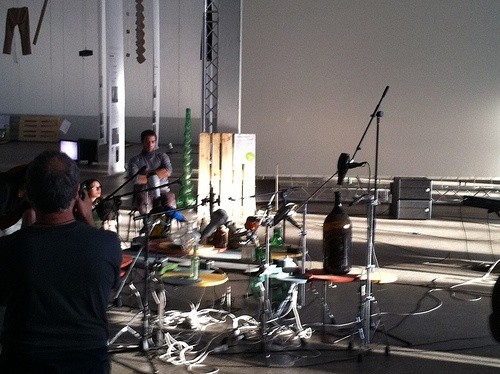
390, 175, 432, 220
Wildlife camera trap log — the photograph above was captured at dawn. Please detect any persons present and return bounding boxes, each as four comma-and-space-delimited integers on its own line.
489, 276, 500, 343
0, 148, 122, 374
128, 130, 173, 237
73, 178, 108, 229
200, 216, 260, 247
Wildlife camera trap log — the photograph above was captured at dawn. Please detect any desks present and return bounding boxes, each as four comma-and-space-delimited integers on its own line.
157, 270, 233, 347
276, 262, 399, 343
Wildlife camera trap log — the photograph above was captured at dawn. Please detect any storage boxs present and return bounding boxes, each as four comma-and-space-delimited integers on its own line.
390, 177, 432, 221
9, 114, 58, 143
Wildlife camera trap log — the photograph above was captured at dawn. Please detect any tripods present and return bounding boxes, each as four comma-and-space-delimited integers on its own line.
108, 85, 412, 367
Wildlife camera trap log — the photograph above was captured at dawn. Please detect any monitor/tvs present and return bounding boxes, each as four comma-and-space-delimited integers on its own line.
57, 138, 97, 164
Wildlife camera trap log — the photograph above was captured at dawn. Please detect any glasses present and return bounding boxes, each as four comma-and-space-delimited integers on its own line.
89, 186, 101, 190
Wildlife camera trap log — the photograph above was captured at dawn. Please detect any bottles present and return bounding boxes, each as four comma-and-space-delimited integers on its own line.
324, 191, 353, 275
190, 246, 200, 279
271, 227, 282, 246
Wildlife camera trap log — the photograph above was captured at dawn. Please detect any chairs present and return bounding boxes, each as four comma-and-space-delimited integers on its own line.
120, 250, 134, 280
126, 183, 146, 241
